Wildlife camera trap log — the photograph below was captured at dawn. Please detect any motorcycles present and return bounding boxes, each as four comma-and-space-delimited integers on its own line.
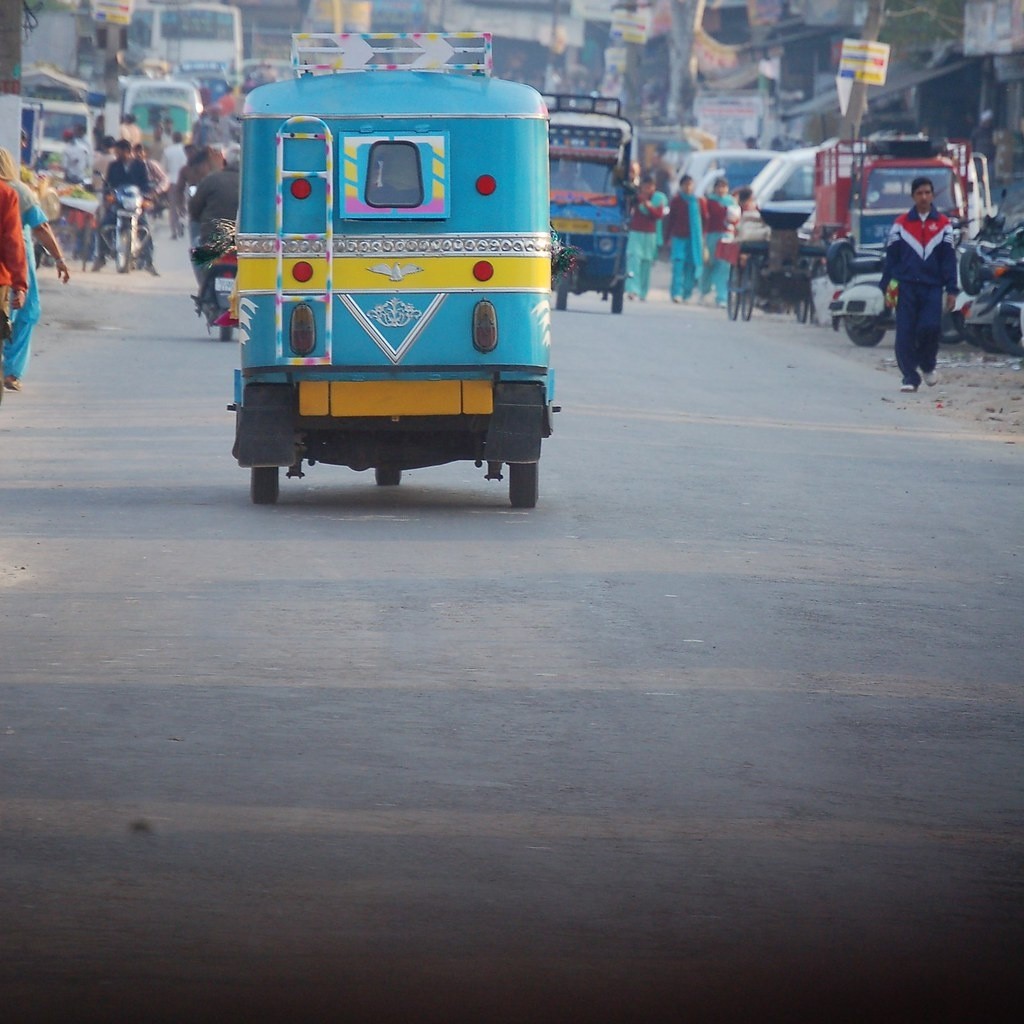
541, 93, 638, 314
94, 169, 156, 274
638, 126, 1024, 356
228, 32, 561, 507
122, 80, 203, 156
189, 185, 238, 341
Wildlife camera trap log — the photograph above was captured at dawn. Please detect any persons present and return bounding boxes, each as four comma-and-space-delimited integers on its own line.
58, 108, 169, 275
667, 174, 709, 304
0, 131, 70, 401
182, 84, 243, 305
162, 129, 191, 239
881, 177, 959, 393
624, 177, 670, 302
697, 177, 763, 307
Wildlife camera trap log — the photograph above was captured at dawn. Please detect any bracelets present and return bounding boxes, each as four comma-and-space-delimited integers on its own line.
56, 258, 65, 263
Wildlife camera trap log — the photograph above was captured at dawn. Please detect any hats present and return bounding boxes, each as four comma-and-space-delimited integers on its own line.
63, 128, 73, 140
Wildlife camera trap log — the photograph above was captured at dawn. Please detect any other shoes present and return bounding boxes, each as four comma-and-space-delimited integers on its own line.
901, 385, 918, 392
92, 258, 107, 272
4, 376, 23, 391
145, 264, 159, 277
923, 371, 938, 387
